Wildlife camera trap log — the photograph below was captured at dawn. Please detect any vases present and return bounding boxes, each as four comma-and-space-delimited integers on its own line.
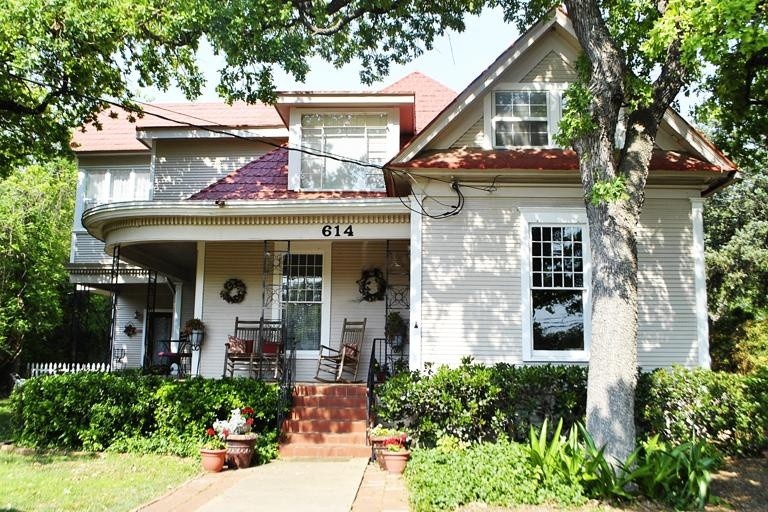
225, 437, 256, 471
200, 448, 225, 473
385, 454, 411, 475
370, 438, 390, 471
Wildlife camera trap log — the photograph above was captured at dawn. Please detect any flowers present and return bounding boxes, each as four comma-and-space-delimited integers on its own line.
365, 424, 393, 436
206, 427, 228, 452
212, 407, 256, 436
381, 431, 411, 453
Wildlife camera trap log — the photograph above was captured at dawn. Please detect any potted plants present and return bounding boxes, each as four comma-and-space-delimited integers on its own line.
382, 311, 408, 348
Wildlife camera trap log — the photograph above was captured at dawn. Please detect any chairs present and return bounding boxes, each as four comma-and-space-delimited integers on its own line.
157, 314, 291, 386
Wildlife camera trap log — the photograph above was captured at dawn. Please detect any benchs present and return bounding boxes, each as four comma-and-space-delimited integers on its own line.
310, 314, 370, 383
